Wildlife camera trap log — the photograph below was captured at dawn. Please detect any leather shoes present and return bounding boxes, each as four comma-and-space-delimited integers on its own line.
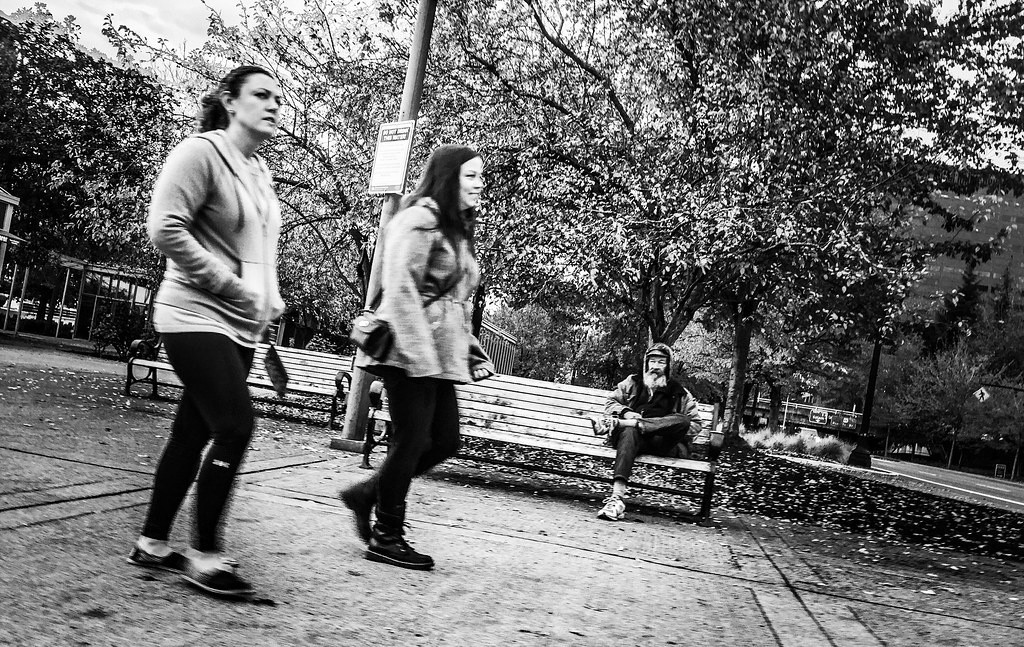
366, 526, 435, 569
339, 479, 374, 543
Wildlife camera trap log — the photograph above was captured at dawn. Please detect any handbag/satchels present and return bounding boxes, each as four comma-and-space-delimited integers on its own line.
349, 306, 395, 363
263, 343, 290, 400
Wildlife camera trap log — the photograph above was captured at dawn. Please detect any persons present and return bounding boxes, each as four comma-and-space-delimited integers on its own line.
127, 64, 287, 594
590, 343, 703, 522
336, 143, 496, 571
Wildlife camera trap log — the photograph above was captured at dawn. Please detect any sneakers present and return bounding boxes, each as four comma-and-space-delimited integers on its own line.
590, 416, 618, 436
596, 494, 628, 521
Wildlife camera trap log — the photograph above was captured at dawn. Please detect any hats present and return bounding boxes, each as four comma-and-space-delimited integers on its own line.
647, 349, 669, 357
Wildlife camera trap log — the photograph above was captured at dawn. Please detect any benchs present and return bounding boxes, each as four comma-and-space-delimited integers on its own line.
124, 330, 357, 430
358, 371, 723, 528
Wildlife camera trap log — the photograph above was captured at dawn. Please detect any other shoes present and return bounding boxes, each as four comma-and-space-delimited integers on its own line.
180, 559, 257, 596
126, 543, 190, 575
